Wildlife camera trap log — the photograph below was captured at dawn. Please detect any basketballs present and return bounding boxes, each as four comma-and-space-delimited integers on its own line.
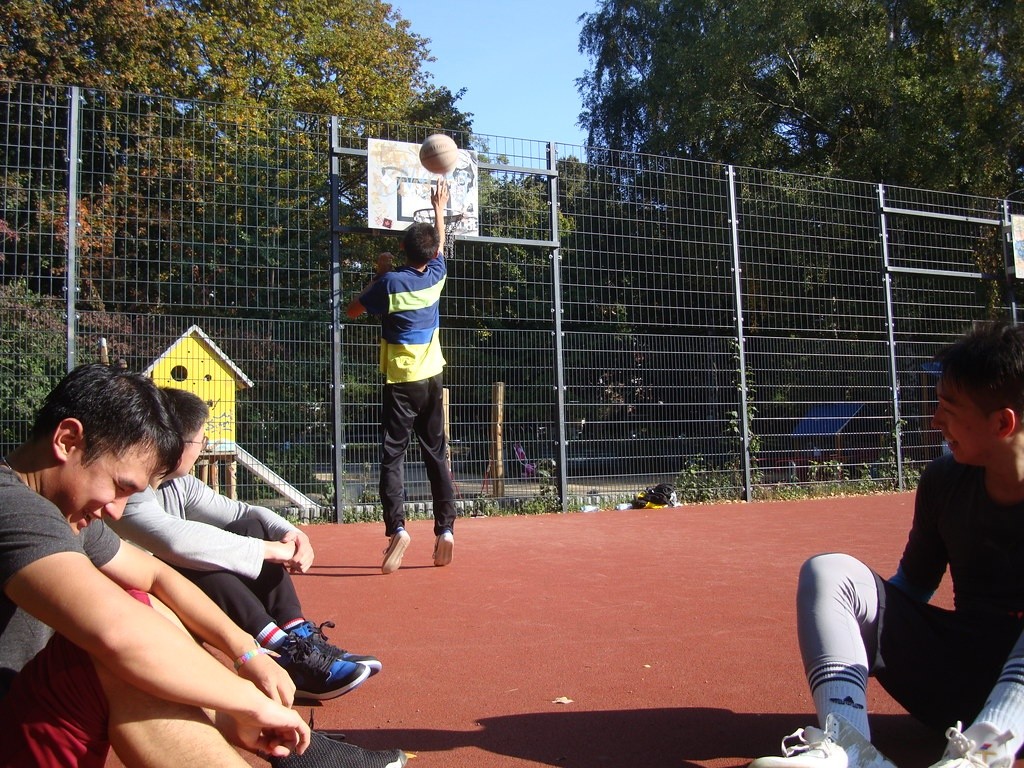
419, 133, 460, 175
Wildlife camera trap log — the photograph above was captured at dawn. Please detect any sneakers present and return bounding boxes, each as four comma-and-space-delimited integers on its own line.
928, 720, 1014, 768
432, 529, 454, 566
381, 526, 411, 573
282, 621, 382, 678
749, 712, 897, 768
269, 630, 372, 700
264, 707, 407, 768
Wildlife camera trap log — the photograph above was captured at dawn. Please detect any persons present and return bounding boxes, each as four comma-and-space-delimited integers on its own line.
0, 363, 408, 768
346, 178, 456, 573
104, 387, 382, 700
747, 322, 1024, 768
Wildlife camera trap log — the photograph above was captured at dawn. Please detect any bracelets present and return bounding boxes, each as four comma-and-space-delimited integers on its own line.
234, 639, 281, 670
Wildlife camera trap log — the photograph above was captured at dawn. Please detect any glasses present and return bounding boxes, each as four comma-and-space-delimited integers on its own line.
179, 436, 209, 452
